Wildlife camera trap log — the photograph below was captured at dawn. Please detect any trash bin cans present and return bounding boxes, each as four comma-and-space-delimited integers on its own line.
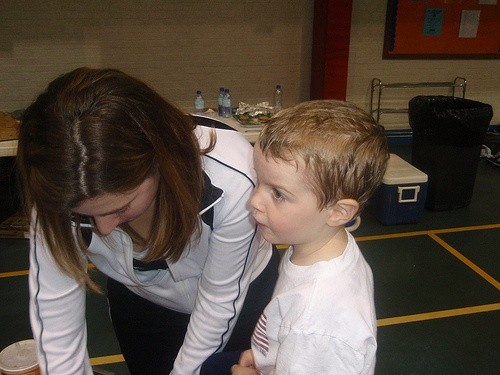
409, 96, 493, 211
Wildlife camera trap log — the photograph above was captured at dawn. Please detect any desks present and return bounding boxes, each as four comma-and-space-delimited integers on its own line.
0, 107, 275, 157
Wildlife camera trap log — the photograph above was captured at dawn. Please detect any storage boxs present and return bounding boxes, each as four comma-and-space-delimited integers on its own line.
367, 152, 428, 226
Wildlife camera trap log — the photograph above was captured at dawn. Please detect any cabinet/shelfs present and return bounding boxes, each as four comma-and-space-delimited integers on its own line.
370, 75, 466, 138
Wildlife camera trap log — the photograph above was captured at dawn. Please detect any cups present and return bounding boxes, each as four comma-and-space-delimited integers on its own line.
0, 339, 40, 375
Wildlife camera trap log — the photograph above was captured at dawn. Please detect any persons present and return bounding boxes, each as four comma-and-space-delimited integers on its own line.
17, 67, 280, 375
232, 100, 390, 375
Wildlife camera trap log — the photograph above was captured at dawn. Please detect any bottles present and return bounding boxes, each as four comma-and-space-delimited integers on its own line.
222, 89, 232, 118
195, 91, 204, 115
217, 88, 225, 116
273, 85, 282, 112
